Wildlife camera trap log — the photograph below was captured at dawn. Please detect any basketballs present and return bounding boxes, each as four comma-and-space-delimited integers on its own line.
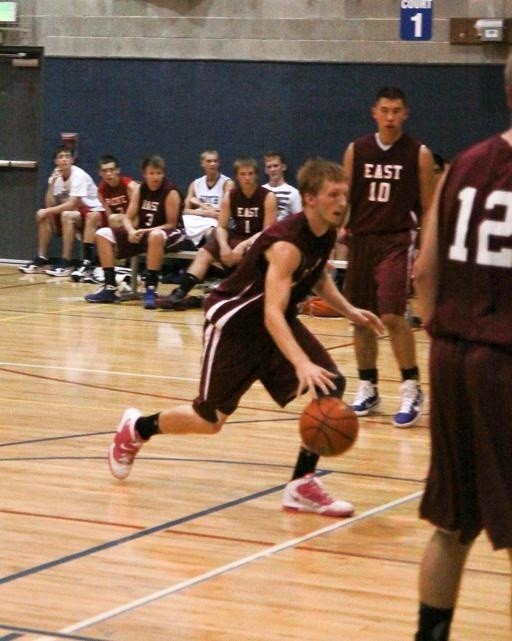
300, 397, 358, 456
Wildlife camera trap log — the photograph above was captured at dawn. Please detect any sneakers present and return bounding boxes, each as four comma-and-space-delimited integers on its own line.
392, 380, 426, 430
108, 407, 150, 480
42, 262, 72, 276
85, 284, 121, 303
143, 286, 156, 309
154, 289, 183, 309
349, 382, 382, 417
174, 295, 202, 311
70, 260, 92, 281
281, 473, 357, 518
20, 257, 52, 273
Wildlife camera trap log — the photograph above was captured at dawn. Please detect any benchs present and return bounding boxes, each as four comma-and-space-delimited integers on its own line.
125, 246, 347, 316
